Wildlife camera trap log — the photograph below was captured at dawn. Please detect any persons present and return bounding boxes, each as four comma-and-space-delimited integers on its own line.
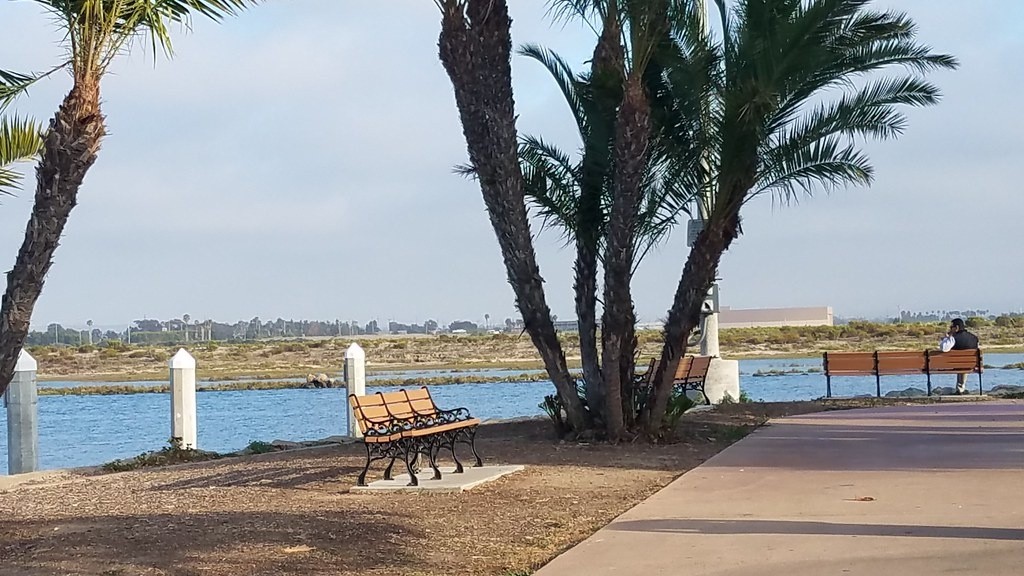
940, 318, 979, 395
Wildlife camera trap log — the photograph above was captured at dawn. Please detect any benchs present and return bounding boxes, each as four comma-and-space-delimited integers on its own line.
822, 349, 984, 398
348, 385, 483, 486
632, 355, 712, 414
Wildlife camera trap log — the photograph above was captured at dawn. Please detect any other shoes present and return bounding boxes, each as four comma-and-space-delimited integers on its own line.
956, 389, 969, 395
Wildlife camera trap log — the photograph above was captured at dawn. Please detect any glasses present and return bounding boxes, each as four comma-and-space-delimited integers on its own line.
950, 325, 956, 330
